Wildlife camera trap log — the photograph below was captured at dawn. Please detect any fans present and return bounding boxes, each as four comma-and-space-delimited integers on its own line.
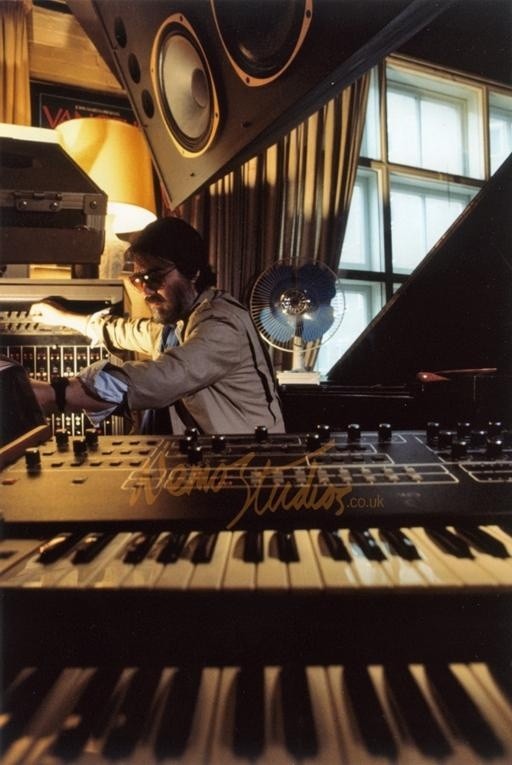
248, 255, 348, 386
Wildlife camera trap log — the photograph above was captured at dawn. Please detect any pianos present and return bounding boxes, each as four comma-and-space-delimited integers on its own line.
1, 524, 512, 765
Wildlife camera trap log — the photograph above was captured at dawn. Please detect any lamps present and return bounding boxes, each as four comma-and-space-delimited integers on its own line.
54, 116, 158, 279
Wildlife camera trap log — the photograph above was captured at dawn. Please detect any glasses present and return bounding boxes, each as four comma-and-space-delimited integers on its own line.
128, 265, 178, 292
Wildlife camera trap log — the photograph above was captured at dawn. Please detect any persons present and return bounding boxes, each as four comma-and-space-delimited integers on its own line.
28, 217, 286, 436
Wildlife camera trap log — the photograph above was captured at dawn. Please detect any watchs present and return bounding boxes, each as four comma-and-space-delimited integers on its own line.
51, 376, 70, 407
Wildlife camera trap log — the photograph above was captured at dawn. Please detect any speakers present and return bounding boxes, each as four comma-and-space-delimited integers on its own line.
86, 1, 456, 214
